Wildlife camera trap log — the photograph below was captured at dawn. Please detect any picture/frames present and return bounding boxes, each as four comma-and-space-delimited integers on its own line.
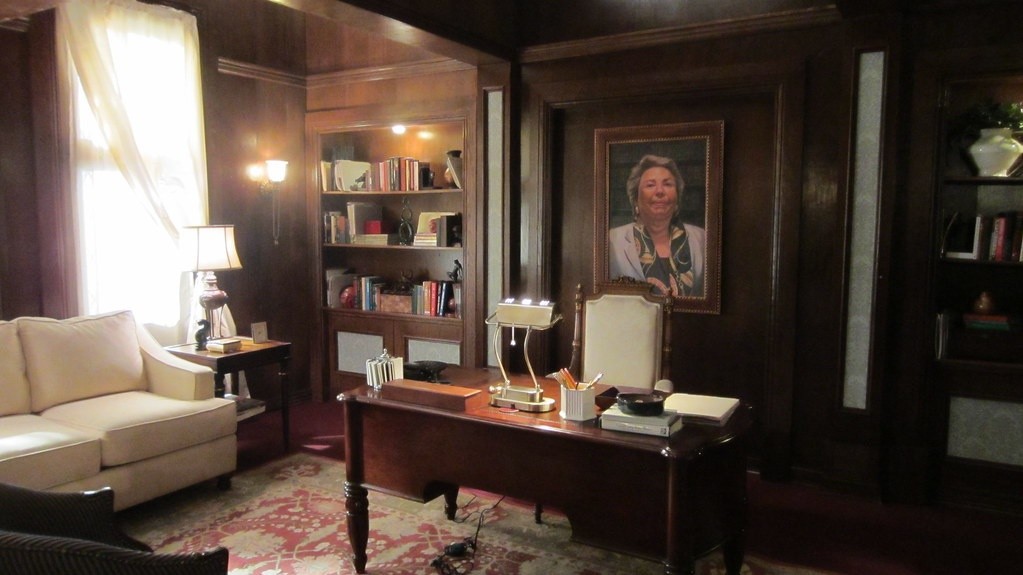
594, 119, 723, 316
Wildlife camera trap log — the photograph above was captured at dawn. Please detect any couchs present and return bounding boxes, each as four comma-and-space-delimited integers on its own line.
0, 310, 238, 512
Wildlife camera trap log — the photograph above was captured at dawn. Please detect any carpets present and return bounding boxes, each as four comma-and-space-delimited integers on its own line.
118, 451, 840, 575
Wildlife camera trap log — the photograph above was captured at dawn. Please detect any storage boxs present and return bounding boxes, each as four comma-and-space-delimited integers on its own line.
381, 379, 482, 412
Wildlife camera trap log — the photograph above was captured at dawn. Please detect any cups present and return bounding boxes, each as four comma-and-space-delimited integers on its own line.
559, 382, 597, 421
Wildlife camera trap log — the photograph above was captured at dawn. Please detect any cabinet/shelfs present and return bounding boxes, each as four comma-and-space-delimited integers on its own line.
305, 94, 477, 406
919, 72, 1023, 516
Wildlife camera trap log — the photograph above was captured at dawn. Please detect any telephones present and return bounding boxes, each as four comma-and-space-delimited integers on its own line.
403, 362, 430, 381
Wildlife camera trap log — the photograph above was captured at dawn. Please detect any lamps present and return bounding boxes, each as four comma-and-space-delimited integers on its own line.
483, 299, 563, 414
260, 160, 288, 246
181, 225, 242, 341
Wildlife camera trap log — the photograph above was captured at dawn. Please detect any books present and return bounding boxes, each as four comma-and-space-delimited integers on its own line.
326, 267, 366, 308
413, 212, 462, 247
663, 394, 740, 427
963, 314, 1010, 330
934, 313, 950, 360
356, 234, 401, 245
372, 156, 433, 191
945, 216, 1023, 262
324, 201, 383, 244
353, 275, 393, 312
447, 156, 462, 188
320, 160, 371, 192
224, 392, 266, 421
452, 284, 461, 318
599, 403, 685, 439
412, 280, 454, 317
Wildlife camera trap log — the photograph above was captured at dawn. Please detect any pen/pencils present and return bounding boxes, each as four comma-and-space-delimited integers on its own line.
590, 373, 604, 387
561, 368, 576, 389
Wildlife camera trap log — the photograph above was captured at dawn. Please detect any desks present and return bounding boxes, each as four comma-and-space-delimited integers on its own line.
336, 360, 763, 575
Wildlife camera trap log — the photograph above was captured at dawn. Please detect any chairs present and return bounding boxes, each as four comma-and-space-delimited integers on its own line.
534, 278, 675, 523
0, 479, 229, 575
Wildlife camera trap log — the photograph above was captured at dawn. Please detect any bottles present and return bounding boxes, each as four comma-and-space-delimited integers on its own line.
973, 290, 992, 314
445, 150, 462, 189
340, 285, 355, 309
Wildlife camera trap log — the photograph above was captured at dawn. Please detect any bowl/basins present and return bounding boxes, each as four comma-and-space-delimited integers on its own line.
616, 393, 664, 416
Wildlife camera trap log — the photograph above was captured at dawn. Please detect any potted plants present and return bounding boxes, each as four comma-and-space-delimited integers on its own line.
941, 94, 1023, 178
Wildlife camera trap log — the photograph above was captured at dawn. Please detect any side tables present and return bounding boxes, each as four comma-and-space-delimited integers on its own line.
164, 334, 293, 451
